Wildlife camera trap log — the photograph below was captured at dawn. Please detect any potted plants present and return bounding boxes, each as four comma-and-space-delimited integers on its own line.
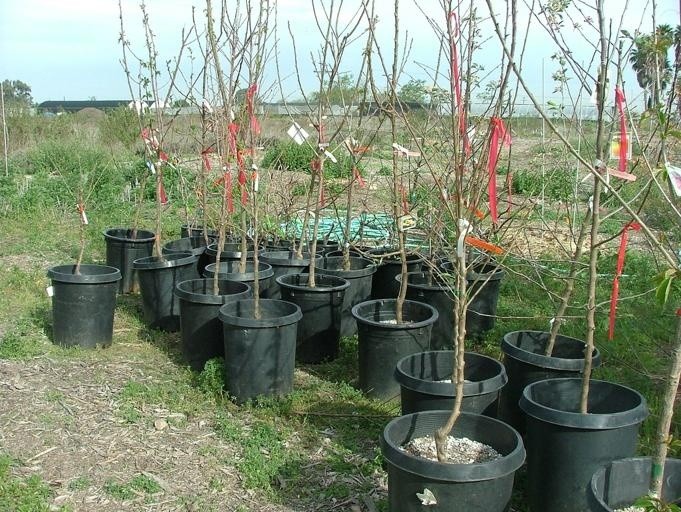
32, 121, 122, 351
